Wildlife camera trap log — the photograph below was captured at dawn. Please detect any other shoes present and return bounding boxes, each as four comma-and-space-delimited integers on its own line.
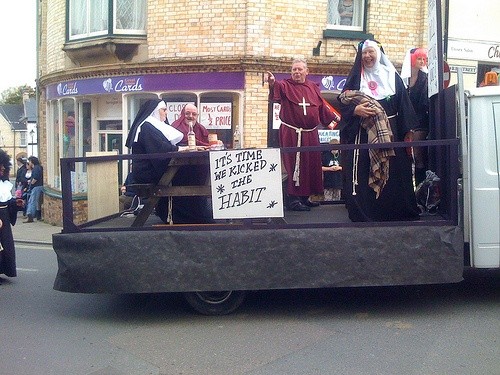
22, 217, 33, 223
292, 203, 311, 211
305, 200, 320, 207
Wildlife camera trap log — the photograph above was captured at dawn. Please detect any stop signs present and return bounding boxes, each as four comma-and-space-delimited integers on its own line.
443, 62, 451, 88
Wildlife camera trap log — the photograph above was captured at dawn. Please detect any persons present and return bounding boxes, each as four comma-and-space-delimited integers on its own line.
337, 39, 422, 222
0, 149, 44, 279
119, 173, 139, 212
399, 48, 428, 187
321, 139, 343, 201
265, 59, 336, 212
170, 103, 210, 185
125, 98, 189, 224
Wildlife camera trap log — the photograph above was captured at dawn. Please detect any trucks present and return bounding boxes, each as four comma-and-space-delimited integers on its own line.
50, 68, 500, 316
